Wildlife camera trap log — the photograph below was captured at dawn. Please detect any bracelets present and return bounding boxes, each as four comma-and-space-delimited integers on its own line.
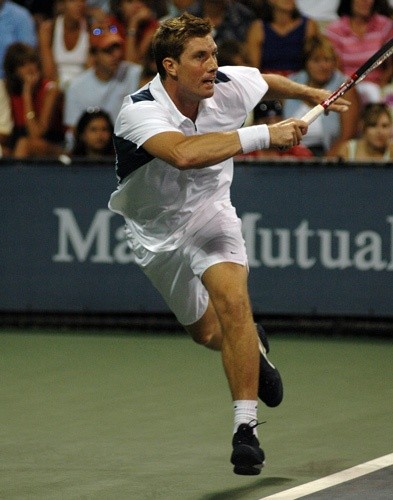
238, 123, 272, 155
125, 30, 140, 37
25, 111, 37, 121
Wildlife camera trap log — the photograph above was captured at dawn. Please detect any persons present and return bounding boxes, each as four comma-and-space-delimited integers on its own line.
201, 1, 265, 70
247, 1, 318, 70
61, 28, 144, 161
325, 103, 393, 164
233, 99, 314, 161
0, 80, 14, 160
38, 0, 91, 113
159, 1, 201, 23
104, 0, 161, 86
69, 107, 114, 162
5, 42, 65, 161
280, 32, 360, 162
327, 0, 393, 104
0, 0, 38, 79
108, 12, 352, 475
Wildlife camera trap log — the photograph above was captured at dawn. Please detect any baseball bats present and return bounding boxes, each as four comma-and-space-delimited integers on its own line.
279, 39, 393, 151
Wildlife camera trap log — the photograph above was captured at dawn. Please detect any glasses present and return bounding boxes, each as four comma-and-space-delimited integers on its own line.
359, 100, 389, 115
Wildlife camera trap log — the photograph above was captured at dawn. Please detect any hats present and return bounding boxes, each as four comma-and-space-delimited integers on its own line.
88, 19, 123, 49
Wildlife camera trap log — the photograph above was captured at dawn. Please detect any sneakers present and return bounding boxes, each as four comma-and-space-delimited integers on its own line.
250, 320, 283, 408
229, 419, 265, 476
